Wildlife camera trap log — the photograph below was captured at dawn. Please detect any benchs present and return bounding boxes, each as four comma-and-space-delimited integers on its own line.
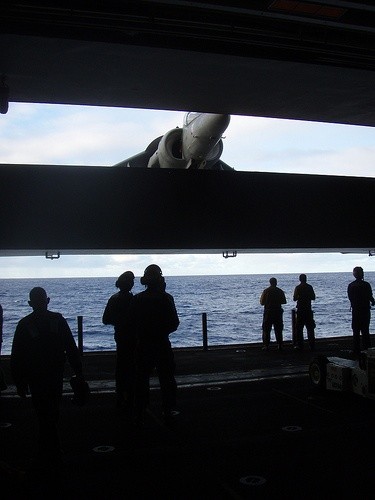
0, 365, 310, 397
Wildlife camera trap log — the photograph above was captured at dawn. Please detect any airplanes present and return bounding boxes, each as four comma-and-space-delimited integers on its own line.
113, 112, 238, 171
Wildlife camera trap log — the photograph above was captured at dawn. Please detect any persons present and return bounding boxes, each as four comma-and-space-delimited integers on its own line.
293, 274, 315, 349
103, 263, 181, 406
260, 277, 287, 350
11, 287, 90, 437
348, 267, 375, 349
